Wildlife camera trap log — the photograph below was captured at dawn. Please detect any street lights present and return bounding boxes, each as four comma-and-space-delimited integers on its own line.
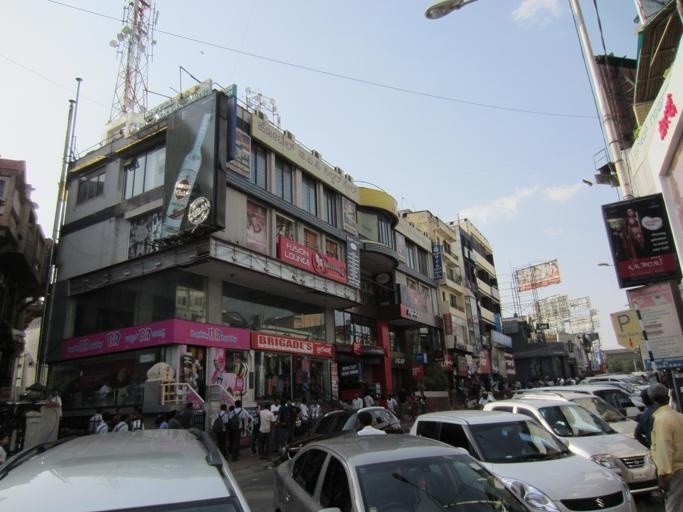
424, 0, 634, 202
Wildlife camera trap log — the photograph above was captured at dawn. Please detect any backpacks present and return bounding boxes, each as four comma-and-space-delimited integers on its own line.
227, 409, 243, 433
213, 412, 227, 434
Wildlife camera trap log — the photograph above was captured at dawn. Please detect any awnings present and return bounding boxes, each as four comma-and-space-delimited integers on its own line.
74, 358, 136, 391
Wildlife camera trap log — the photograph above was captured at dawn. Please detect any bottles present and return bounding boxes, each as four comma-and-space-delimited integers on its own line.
159, 112, 213, 240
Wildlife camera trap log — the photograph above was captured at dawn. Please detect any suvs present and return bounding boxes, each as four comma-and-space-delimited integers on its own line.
0, 427, 252, 512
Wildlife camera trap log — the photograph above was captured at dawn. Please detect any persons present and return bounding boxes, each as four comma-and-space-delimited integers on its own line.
462, 371, 583, 411
0, 435, 9, 466
212, 397, 322, 462
89, 380, 193, 434
50, 388, 63, 416
352, 388, 427, 435
634, 382, 683, 512
